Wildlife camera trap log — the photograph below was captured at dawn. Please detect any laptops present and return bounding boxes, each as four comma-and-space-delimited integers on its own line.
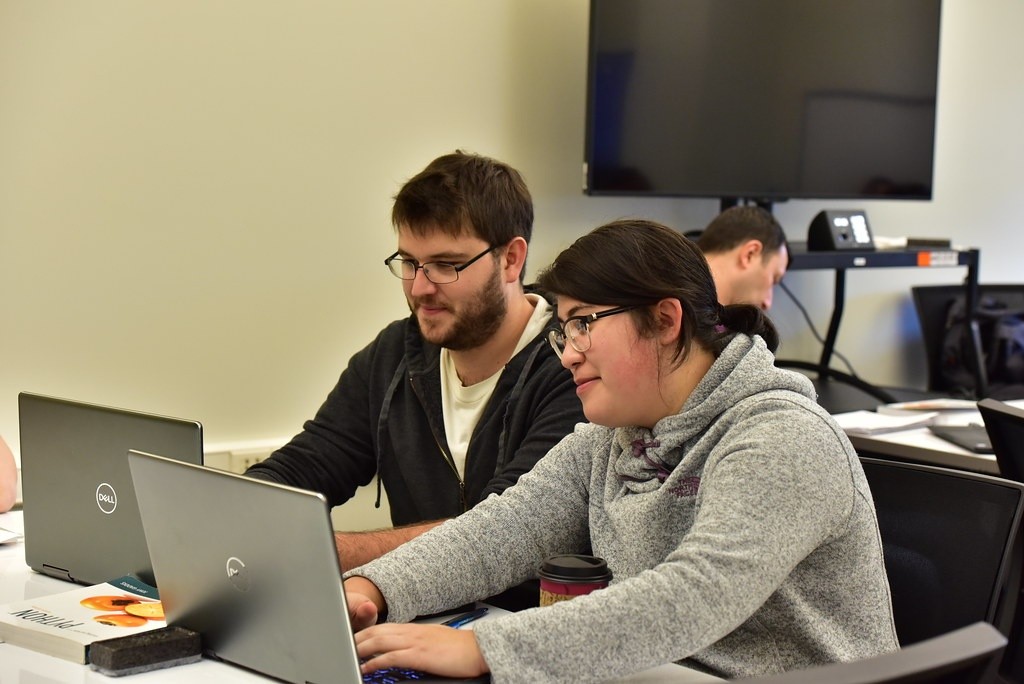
127, 448, 513, 684
18, 392, 203, 586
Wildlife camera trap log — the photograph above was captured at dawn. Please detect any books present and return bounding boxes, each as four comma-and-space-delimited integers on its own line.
0, 576, 167, 665
877, 398, 979, 416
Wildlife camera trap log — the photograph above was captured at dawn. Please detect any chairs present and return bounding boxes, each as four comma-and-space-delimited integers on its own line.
729, 456, 1024, 684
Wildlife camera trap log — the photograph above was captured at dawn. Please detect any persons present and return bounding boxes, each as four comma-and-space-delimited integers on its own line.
696, 206, 791, 309
241, 151, 591, 570
340, 220, 900, 684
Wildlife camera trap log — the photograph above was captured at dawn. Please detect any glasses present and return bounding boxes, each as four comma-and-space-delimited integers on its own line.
385, 242, 504, 284
544, 306, 637, 360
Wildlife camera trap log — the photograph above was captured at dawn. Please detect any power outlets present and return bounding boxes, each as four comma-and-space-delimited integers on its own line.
231, 451, 270, 475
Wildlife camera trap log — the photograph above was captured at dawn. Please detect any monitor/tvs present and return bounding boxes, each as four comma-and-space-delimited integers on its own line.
582, 0, 943, 200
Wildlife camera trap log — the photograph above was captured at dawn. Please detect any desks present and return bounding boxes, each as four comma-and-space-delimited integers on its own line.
830, 398, 1024, 475
